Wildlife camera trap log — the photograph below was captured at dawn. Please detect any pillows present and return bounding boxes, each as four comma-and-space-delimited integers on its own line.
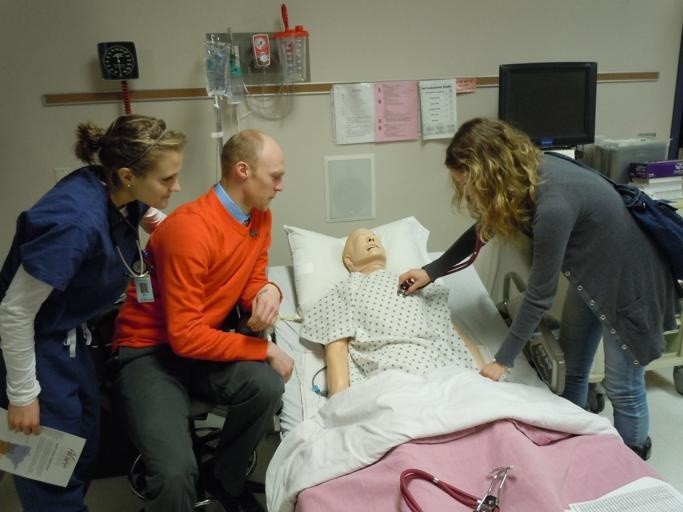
280, 215, 434, 314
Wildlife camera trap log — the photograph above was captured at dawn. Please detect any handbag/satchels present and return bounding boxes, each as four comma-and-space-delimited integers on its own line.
616, 183, 683, 279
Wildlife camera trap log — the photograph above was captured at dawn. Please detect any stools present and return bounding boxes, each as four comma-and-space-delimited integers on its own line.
123, 401, 264, 512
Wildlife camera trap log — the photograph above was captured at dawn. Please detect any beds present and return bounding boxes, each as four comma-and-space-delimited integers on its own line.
255, 252, 683, 509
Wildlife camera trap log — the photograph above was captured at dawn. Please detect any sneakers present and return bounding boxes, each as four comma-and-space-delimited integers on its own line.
627, 438, 652, 461
200, 458, 265, 512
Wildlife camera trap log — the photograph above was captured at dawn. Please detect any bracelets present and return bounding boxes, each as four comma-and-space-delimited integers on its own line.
492, 358, 512, 377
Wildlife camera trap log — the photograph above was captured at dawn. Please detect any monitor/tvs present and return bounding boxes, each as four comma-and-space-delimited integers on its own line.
498, 61, 598, 151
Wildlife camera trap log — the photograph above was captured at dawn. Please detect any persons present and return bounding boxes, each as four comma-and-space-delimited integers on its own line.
300, 226, 488, 399
111, 129, 294, 511
398, 117, 683, 460
0, 114, 186, 512
